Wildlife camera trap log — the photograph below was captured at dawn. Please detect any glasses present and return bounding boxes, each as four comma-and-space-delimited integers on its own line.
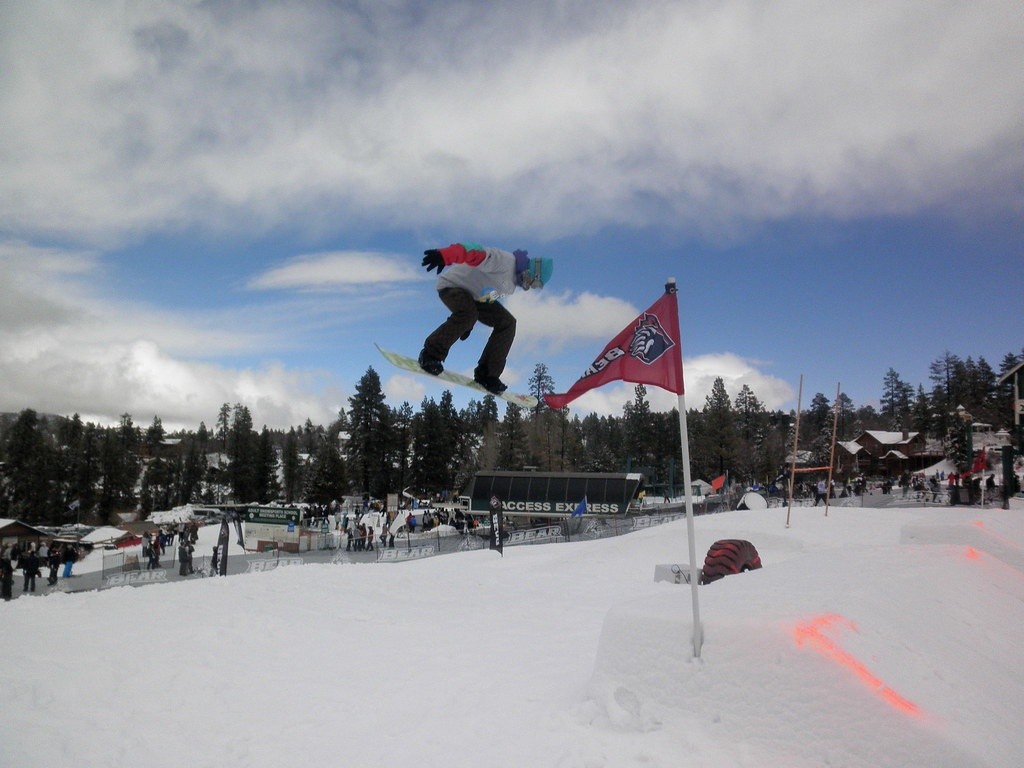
532, 258, 544, 290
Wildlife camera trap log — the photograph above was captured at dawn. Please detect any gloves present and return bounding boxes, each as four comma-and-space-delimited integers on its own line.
460, 326, 473, 340
422, 249, 445, 275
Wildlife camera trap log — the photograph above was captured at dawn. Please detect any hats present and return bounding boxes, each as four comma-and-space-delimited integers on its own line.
527, 256, 553, 286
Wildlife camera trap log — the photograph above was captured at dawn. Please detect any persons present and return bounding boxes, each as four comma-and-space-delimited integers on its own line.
792, 470, 1021, 509
418, 242, 555, 393
0, 487, 671, 601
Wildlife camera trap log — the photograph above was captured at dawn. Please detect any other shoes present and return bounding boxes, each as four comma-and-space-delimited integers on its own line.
418, 349, 444, 375
475, 373, 507, 393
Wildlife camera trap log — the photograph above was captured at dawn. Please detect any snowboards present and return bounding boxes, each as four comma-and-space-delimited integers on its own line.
373, 342, 539, 408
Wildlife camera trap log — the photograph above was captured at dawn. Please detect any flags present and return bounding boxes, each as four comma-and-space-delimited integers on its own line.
544, 282, 684, 410
712, 474, 725, 491
962, 447, 986, 479
